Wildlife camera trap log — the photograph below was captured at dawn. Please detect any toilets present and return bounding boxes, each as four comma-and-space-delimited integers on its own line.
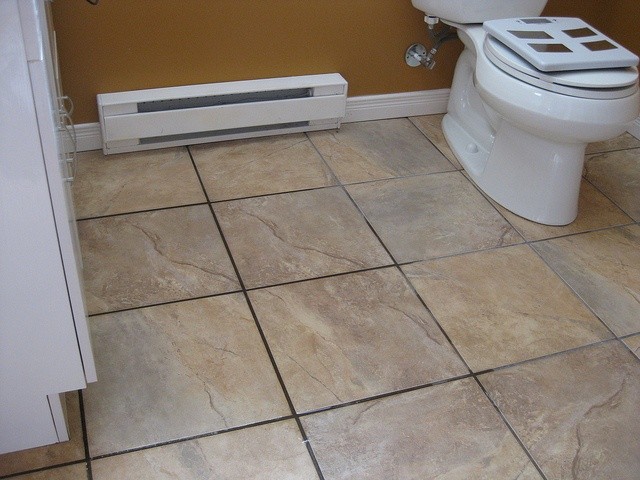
412, 0, 640, 226
